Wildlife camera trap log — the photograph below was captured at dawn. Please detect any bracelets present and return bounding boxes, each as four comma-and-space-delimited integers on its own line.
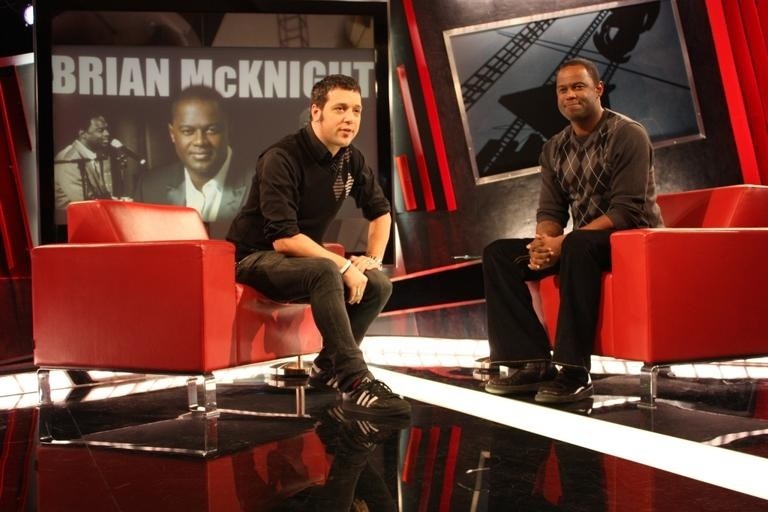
340, 259, 353, 274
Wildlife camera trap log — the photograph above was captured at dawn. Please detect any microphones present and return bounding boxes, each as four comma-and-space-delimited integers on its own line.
111, 139, 147, 165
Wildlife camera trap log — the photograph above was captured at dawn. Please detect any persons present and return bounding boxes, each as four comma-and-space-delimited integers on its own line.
484, 57, 666, 404
224, 74, 410, 417
136, 83, 256, 237
493, 398, 605, 511
48, 108, 134, 227
313, 404, 399, 511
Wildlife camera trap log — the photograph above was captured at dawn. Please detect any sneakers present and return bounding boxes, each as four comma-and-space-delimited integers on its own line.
561, 399, 594, 416
485, 362, 559, 395
308, 362, 412, 415
534, 366, 594, 403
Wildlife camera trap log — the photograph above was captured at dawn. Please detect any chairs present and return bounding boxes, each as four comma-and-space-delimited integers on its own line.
473, 184, 768, 410
31, 200, 345, 457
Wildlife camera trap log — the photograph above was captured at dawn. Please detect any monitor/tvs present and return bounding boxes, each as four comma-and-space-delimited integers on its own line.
33, 0, 397, 268
